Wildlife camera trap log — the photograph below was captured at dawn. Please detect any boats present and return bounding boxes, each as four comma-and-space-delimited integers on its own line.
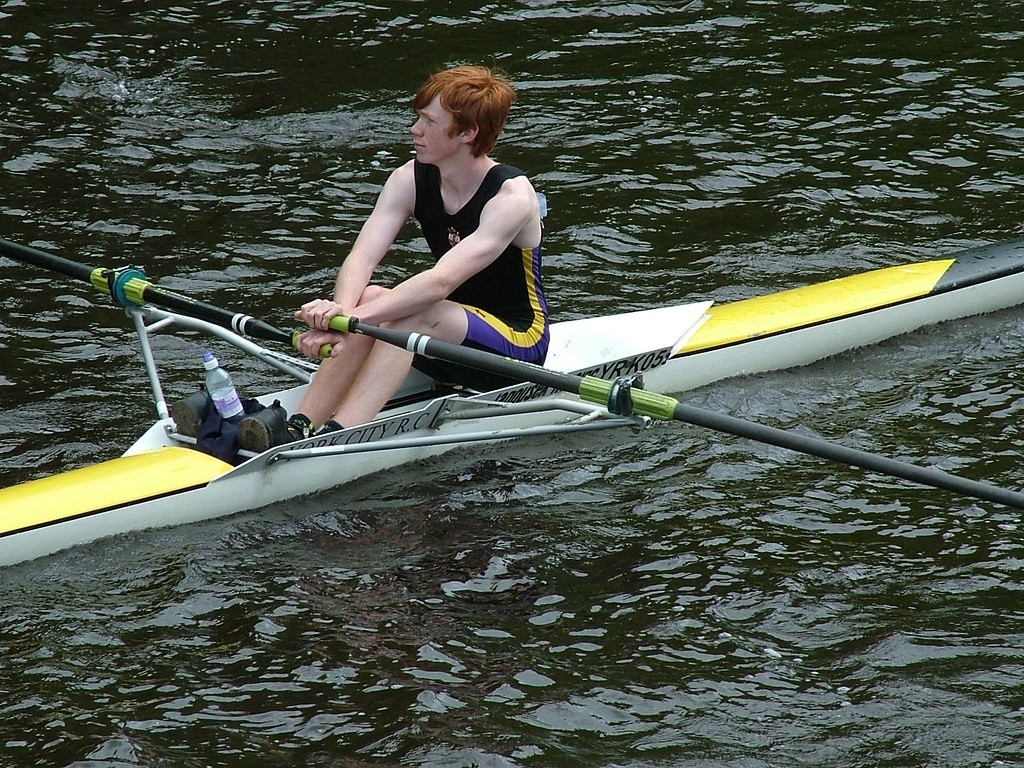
0, 237, 1023, 573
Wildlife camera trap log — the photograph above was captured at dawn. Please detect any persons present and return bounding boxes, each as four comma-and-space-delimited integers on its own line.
288, 65, 549, 440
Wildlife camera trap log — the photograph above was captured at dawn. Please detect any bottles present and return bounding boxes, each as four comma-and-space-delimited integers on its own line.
203, 353, 245, 418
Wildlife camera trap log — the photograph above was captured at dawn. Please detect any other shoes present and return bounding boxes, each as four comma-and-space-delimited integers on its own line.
171, 384, 209, 437
238, 399, 287, 455
